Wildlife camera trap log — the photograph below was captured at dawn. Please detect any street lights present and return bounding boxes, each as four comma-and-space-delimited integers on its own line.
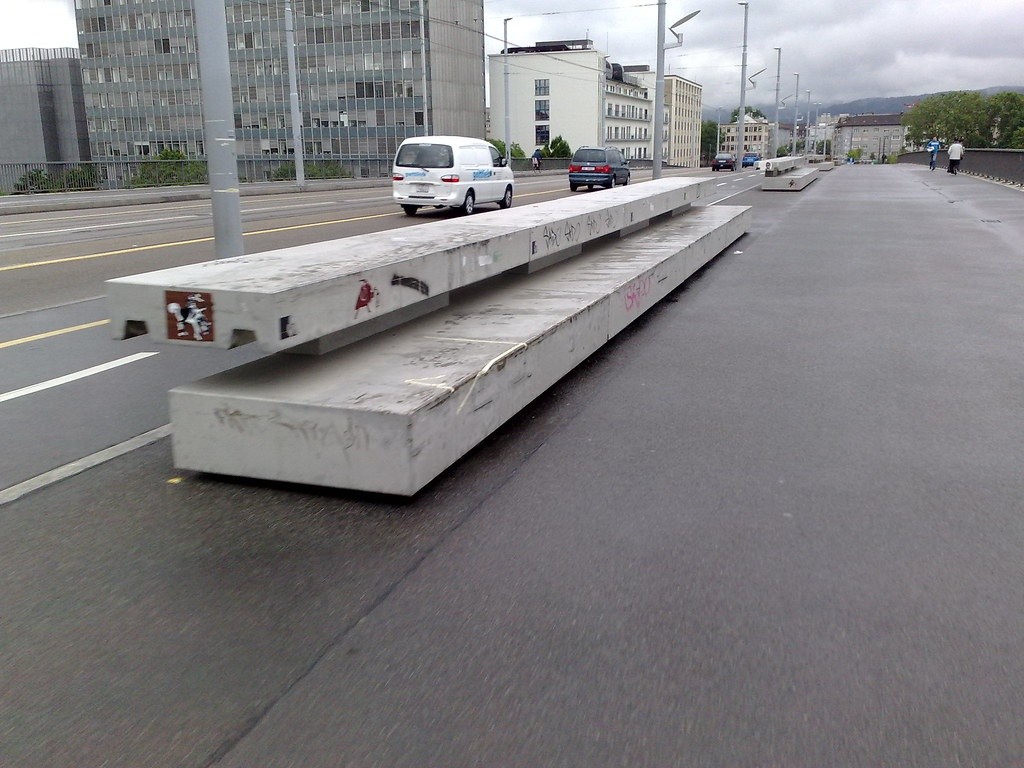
768, 48, 794, 157
812, 101, 822, 155
791, 74, 803, 156
652, 0, 701, 181
823, 112, 830, 162
737, 2, 767, 174
805, 89, 813, 155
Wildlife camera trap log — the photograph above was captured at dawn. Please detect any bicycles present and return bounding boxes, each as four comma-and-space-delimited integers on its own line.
532, 158, 542, 170
950, 160, 960, 175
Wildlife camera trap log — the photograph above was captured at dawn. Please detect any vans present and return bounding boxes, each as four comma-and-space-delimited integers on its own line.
392, 136, 515, 215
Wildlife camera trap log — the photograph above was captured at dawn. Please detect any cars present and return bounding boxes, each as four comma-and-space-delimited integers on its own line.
712, 152, 736, 171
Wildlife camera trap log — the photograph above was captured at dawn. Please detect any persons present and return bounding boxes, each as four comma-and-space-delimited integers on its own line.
534, 149, 542, 170
882, 154, 886, 163
947, 137, 965, 175
927, 136, 941, 170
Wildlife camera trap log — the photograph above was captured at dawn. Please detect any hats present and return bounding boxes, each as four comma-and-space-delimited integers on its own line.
954, 136, 959, 141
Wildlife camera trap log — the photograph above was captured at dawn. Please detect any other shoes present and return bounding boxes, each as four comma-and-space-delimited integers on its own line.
930, 166, 935, 171
950, 169, 958, 175
947, 170, 951, 173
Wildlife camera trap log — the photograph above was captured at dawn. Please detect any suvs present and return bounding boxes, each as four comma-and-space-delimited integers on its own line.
568, 146, 630, 192
742, 152, 760, 168
